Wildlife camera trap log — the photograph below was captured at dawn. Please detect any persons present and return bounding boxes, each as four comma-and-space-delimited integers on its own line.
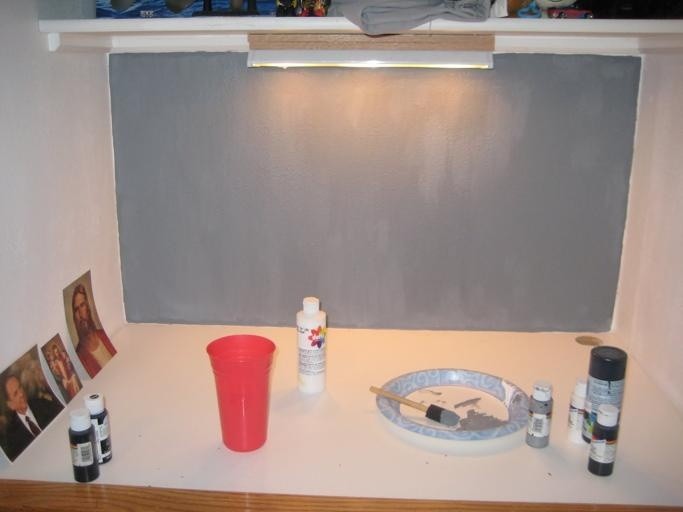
45, 344, 80, 399
1, 372, 59, 461
70, 286, 117, 378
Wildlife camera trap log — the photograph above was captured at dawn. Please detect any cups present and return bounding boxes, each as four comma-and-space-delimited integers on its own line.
206, 334, 277, 453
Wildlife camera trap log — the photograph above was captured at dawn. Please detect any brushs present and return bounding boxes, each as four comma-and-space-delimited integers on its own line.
369, 386, 461, 426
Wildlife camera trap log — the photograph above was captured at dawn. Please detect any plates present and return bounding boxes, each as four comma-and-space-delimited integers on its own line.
375, 368, 529, 441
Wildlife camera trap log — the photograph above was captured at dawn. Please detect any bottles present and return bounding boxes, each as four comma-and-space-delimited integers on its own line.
68, 393, 113, 482
295, 297, 327, 394
527, 347, 628, 477
